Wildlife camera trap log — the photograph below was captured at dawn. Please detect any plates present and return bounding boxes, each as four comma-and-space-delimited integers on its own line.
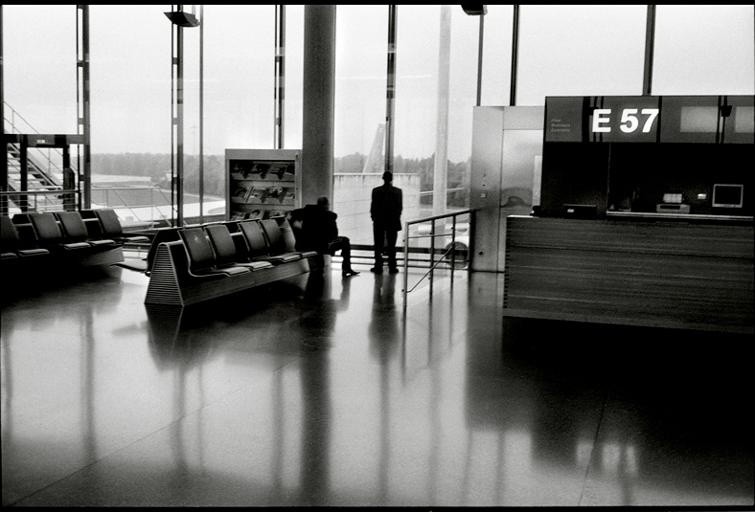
223, 148, 304, 222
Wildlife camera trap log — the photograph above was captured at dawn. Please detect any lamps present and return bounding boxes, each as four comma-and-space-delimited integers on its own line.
0, 208, 127, 272
106, 214, 320, 336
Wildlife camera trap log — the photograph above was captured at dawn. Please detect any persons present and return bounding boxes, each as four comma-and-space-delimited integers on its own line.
370, 171, 402, 274
289, 197, 360, 275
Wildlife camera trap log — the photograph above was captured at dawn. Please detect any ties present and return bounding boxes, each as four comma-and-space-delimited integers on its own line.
712, 183, 744, 209
562, 203, 597, 220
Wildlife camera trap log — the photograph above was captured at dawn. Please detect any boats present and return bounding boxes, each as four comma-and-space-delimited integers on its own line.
163, 12, 199, 27
460, 0, 488, 16
